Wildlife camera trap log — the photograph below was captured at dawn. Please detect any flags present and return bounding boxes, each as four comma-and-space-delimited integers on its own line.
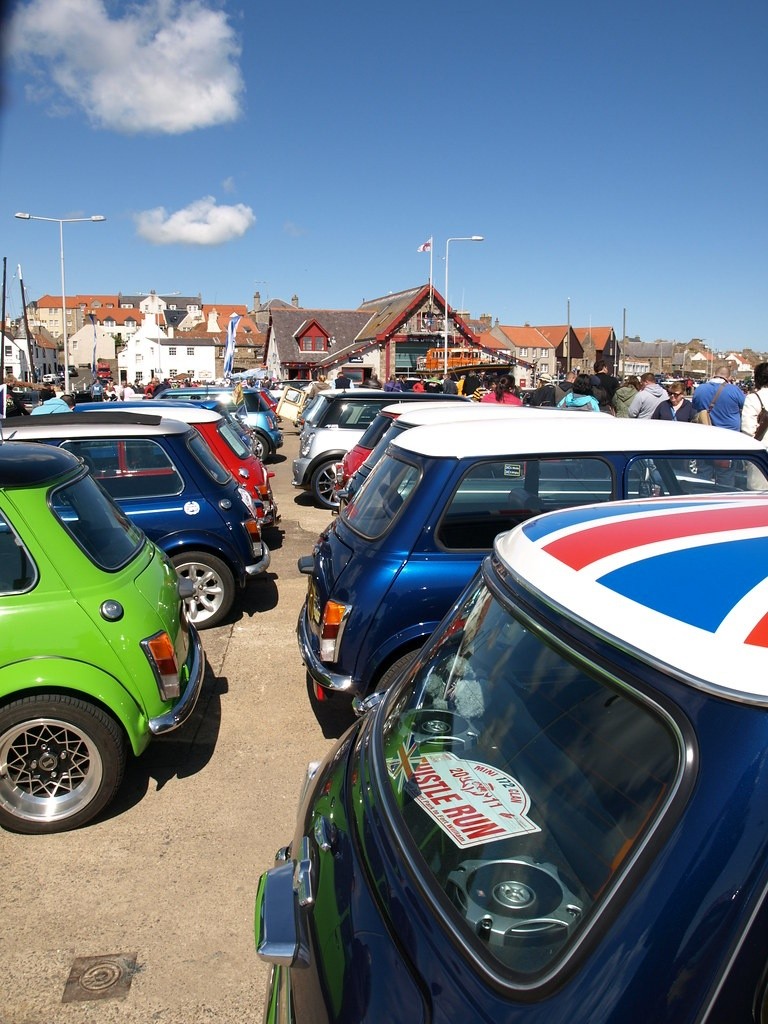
223, 315, 244, 373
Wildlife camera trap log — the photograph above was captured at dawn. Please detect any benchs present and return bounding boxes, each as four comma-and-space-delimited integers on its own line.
377, 482, 485, 548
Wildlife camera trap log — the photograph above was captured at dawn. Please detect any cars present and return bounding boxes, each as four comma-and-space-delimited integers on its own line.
251, 488, 768, 1024
0, 437, 205, 837
1, 361, 768, 717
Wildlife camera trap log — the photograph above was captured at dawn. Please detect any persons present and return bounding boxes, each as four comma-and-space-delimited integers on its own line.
27, 389, 73, 415
692, 366, 748, 488
6, 389, 30, 418
651, 381, 699, 423
628, 373, 670, 420
611, 376, 642, 418
592, 359, 620, 399
740, 362, 768, 491
48, 372, 754, 410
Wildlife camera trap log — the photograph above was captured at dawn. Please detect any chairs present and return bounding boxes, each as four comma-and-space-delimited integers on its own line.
508, 487, 543, 516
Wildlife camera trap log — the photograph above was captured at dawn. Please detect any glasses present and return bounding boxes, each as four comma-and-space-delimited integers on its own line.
668, 391, 683, 397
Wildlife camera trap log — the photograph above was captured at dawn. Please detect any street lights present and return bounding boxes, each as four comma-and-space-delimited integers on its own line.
14, 211, 106, 393
442, 235, 484, 376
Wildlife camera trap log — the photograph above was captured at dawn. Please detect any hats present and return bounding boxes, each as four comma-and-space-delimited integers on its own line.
539, 373, 553, 383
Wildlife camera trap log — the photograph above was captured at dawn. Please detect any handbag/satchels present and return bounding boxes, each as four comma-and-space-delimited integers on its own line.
691, 410, 712, 426
751, 391, 768, 441
